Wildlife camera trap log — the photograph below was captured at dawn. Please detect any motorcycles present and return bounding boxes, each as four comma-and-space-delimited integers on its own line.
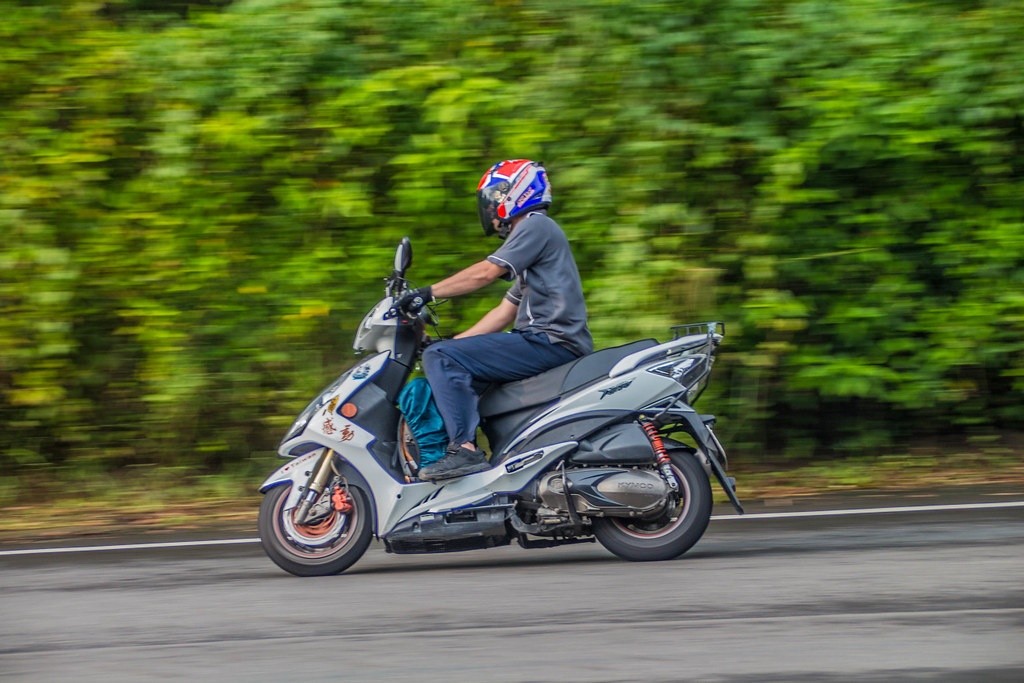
256, 237, 750, 578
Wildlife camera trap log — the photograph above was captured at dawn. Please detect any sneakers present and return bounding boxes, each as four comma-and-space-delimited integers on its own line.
419, 442, 492, 480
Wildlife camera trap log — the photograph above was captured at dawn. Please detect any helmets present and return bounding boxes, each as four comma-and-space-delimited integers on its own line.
478, 159, 553, 237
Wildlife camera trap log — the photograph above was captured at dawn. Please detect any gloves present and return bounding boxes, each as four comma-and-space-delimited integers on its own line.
392, 284, 432, 316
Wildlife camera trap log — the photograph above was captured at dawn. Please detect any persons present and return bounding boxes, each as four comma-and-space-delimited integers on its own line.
388, 158, 594, 481
